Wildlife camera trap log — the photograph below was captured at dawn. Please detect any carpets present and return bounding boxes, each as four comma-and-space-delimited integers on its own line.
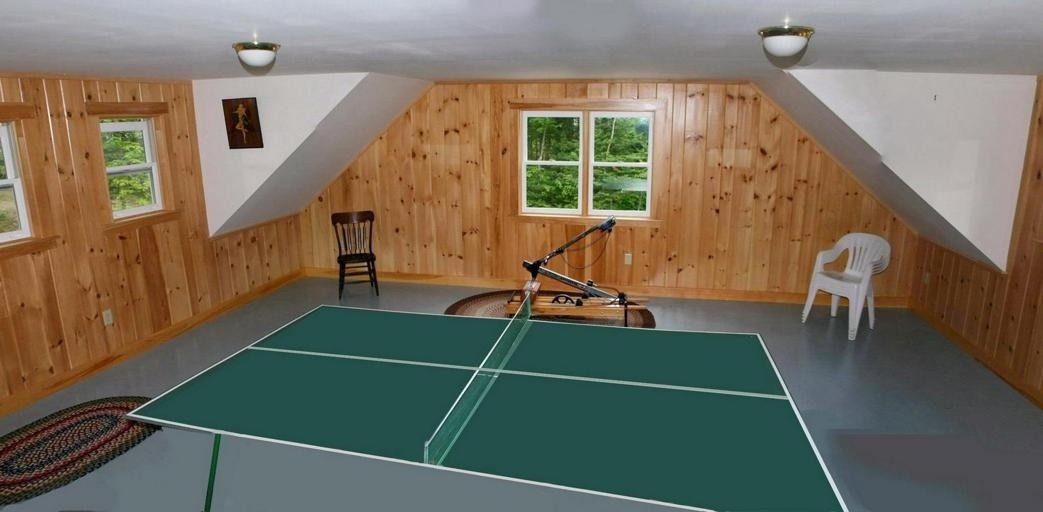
0, 395, 163, 504
444, 288, 656, 328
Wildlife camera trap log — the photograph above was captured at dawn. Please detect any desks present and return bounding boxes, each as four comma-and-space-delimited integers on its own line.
123, 304, 851, 511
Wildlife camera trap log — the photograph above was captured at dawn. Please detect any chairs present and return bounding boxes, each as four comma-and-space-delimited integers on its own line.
801, 231, 891, 341
331, 210, 380, 300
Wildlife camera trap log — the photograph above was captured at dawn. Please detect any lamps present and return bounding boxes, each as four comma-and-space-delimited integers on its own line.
759, 26, 813, 57
232, 42, 281, 66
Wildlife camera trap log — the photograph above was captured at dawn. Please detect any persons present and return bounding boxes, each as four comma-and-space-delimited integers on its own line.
232, 103, 249, 144
